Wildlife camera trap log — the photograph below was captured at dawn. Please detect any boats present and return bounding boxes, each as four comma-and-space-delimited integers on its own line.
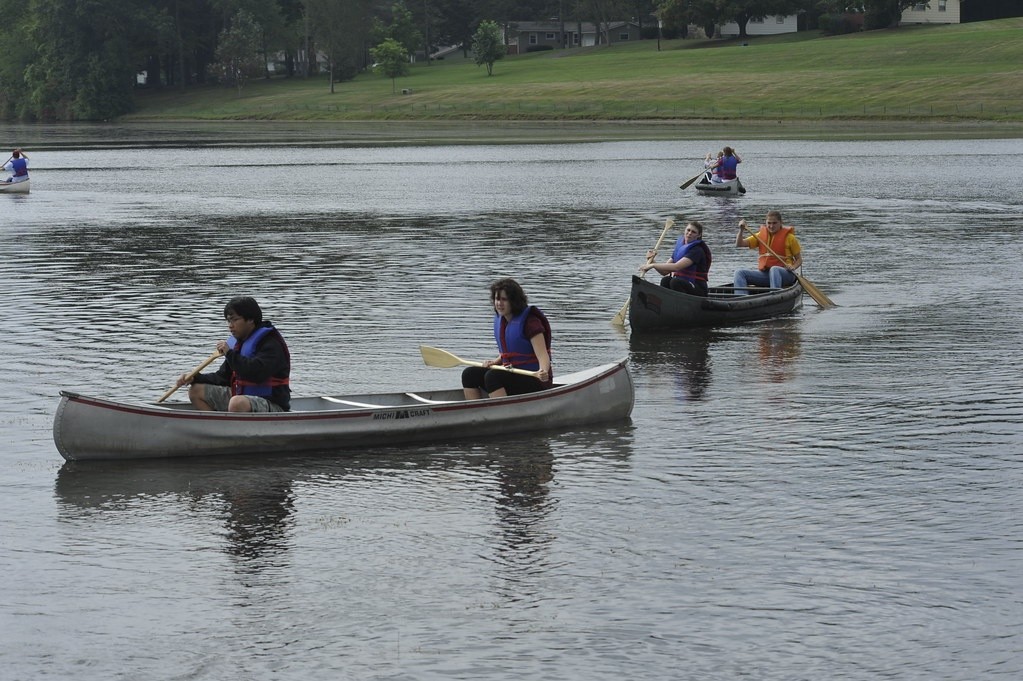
695, 177, 738, 196
629, 264, 803, 331
51, 356, 635, 460
0, 178, 30, 193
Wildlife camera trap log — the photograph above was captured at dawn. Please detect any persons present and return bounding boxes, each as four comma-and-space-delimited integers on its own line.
0, 147, 31, 182
733, 210, 803, 295
461, 279, 554, 401
638, 221, 712, 297
704, 147, 742, 184
176, 296, 291, 412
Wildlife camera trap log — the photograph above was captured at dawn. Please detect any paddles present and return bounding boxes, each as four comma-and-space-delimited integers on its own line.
158, 350, 225, 403
610, 218, 675, 326
710, 157, 746, 194
2, 155, 13, 167
419, 344, 541, 380
742, 224, 837, 310
679, 157, 723, 191
